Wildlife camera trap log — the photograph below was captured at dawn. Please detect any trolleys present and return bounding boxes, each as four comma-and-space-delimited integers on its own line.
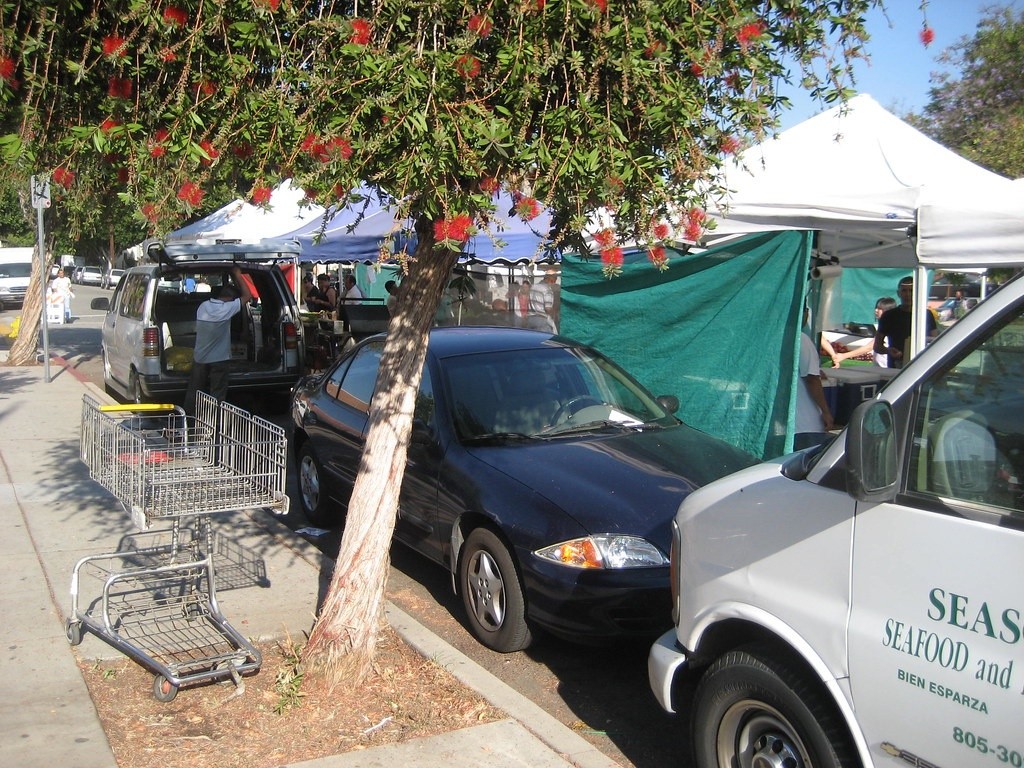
66, 387, 286, 701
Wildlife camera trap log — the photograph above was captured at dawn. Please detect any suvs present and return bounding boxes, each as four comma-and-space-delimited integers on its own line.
101, 269, 125, 290
51, 265, 60, 276
933, 296, 979, 318
79, 266, 103, 286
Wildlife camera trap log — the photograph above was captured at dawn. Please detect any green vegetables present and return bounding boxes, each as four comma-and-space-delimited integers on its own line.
944, 269, 1012, 318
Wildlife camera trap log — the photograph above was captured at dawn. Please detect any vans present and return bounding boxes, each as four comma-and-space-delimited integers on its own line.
0, 247, 34, 310
158, 274, 182, 295
100, 241, 305, 412
649, 272, 1024, 768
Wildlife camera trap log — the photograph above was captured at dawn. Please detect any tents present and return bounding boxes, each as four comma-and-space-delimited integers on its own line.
559, 93, 1024, 462
127, 178, 327, 261
259, 178, 561, 306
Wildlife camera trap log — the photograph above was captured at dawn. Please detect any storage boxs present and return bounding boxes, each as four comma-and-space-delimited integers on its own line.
818, 366, 902, 422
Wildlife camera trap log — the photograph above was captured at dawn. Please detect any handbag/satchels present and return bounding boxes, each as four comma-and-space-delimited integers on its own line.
70, 292, 76, 299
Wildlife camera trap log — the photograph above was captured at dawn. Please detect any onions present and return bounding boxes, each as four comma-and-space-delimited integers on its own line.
820, 342, 873, 361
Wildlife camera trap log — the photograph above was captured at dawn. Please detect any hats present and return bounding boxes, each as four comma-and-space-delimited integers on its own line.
317, 274, 330, 281
385, 280, 396, 293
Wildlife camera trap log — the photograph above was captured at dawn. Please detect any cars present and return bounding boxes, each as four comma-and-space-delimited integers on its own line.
71, 266, 83, 283
287, 327, 767, 653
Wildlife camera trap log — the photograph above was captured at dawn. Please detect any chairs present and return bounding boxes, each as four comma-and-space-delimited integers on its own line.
493, 361, 569, 435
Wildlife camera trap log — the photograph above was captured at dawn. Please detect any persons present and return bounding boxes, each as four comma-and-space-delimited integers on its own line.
492, 270, 560, 335
183, 274, 212, 293
385, 280, 398, 317
952, 291, 964, 317
832, 296, 897, 369
821, 335, 840, 369
874, 276, 938, 369
793, 296, 834, 451
182, 265, 251, 416
51, 269, 73, 323
303, 273, 362, 312
